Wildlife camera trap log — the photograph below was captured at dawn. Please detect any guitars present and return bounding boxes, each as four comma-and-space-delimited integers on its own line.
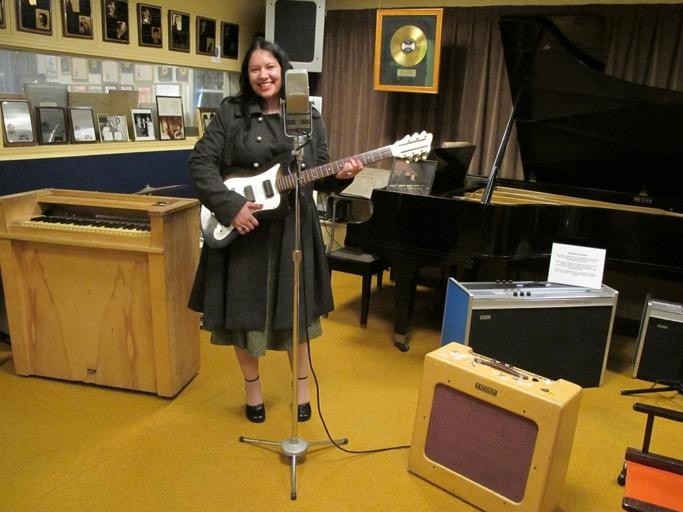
199, 128, 434, 250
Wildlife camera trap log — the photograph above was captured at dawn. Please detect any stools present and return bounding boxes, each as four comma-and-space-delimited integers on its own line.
320, 247, 392, 329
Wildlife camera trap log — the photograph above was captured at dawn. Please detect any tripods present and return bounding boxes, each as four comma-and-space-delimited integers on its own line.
238, 134, 348, 503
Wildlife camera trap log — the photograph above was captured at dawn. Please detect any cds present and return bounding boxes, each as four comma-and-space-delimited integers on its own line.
390, 25, 429, 66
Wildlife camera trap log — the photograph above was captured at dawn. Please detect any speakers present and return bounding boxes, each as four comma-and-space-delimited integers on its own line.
408, 341, 583, 512
266, 0, 326, 73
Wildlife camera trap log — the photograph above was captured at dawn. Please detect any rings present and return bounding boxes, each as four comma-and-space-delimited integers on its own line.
346, 172, 352, 176
241, 225, 246, 231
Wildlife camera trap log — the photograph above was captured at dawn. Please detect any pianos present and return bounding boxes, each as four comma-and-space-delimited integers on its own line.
0, 187, 202, 399
343, 15, 683, 352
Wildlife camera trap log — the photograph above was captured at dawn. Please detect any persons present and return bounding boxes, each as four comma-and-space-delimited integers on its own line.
203, 112, 215, 130
102, 117, 123, 141
136, 116, 152, 137
36, 13, 50, 29
152, 27, 162, 44
80, 16, 91, 35
106, 0, 128, 39
187, 39, 363, 423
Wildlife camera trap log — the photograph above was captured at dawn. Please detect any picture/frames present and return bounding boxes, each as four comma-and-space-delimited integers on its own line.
0, 1, 241, 149
385, 154, 438, 197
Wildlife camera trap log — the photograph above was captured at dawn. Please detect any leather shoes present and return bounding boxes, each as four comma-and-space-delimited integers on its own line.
245, 398, 264, 423
297, 400, 311, 422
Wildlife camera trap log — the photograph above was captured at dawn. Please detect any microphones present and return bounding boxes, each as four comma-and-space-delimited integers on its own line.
281, 69, 312, 135
